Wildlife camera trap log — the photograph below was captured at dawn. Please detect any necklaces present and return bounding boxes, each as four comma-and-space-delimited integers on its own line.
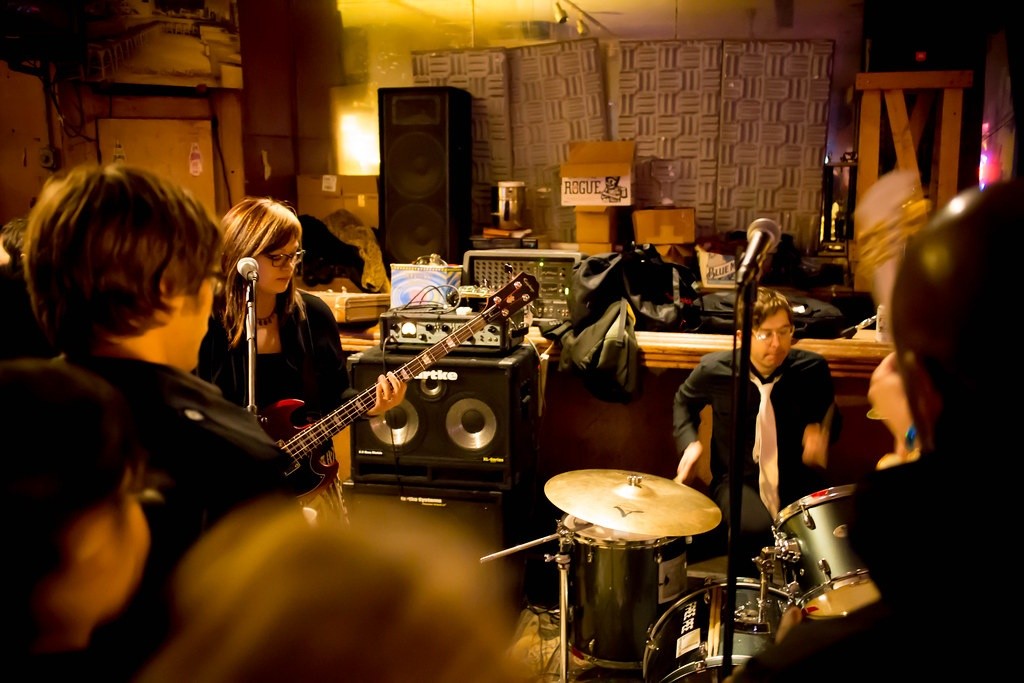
256, 309, 276, 326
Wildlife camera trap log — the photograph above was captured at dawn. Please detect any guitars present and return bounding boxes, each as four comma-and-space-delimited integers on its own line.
257, 269, 540, 503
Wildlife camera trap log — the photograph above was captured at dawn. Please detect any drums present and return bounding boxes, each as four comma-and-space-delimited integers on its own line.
638, 577, 803, 683
554, 513, 687, 670
772, 484, 881, 620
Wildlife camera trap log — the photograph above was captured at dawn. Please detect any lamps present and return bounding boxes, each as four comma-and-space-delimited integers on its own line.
552, 1, 568, 24
576, 14, 592, 36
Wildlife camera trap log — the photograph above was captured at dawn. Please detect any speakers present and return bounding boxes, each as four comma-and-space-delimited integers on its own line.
345, 346, 542, 561
378, 86, 473, 282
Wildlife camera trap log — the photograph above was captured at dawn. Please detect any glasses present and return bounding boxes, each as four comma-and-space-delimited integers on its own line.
752, 325, 795, 341
263, 247, 304, 267
212, 271, 227, 295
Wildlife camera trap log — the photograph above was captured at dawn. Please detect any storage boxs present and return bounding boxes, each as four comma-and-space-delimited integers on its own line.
575, 205, 619, 242
296, 174, 383, 229
560, 139, 636, 206
578, 241, 613, 257
655, 243, 691, 265
632, 207, 696, 245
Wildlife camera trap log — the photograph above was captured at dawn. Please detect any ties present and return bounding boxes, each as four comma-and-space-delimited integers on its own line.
749, 370, 783, 519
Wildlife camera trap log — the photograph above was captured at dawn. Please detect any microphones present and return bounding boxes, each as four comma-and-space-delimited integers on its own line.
237, 257, 260, 282
737, 218, 782, 288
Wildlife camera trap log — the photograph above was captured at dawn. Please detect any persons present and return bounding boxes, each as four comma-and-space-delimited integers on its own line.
671, 287, 842, 565
138, 490, 534, 683
22, 160, 295, 648
0, 359, 150, 683
721, 169, 1024, 683
191, 196, 408, 528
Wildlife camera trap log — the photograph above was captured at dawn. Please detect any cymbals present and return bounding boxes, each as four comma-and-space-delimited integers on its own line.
545, 467, 722, 539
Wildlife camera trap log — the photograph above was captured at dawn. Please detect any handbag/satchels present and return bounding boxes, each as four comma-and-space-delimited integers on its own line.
563, 240, 701, 337
390, 255, 462, 308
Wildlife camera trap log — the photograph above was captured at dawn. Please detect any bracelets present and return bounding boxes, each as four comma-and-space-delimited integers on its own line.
905, 426, 917, 446
